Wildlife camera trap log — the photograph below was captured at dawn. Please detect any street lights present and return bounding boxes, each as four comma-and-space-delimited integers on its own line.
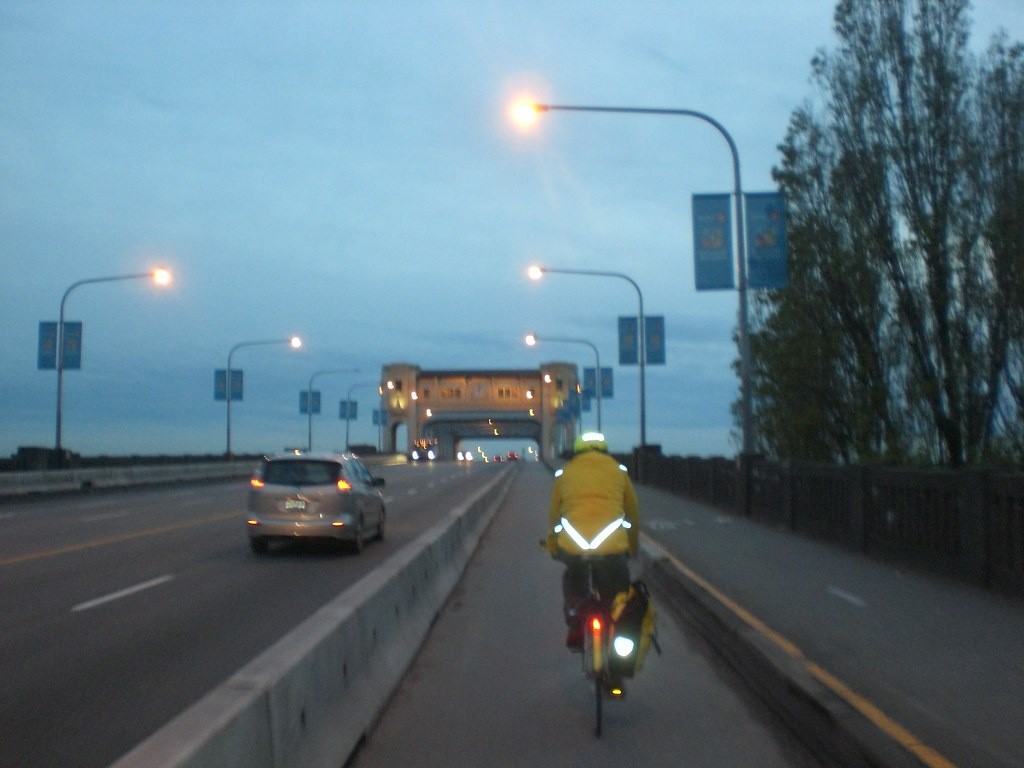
226, 337, 302, 460
509, 100, 752, 514
525, 335, 601, 434
55, 266, 170, 465
307, 364, 360, 453
346, 380, 416, 452
528, 265, 644, 488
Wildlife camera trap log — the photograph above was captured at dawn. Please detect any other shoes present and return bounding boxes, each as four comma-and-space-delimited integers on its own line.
565, 616, 584, 648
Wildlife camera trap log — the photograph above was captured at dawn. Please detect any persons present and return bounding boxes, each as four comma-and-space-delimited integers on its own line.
546, 430, 640, 697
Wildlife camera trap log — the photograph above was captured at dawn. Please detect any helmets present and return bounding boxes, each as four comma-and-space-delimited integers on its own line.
574, 431, 608, 452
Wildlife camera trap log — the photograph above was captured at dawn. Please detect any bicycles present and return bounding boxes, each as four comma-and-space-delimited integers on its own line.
541, 541, 617, 738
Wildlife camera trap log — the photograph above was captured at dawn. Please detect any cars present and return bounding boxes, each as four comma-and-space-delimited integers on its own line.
494, 452, 518, 462
247, 453, 387, 554
412, 443, 439, 461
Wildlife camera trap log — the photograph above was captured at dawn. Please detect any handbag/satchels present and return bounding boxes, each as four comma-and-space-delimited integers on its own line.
606, 580, 662, 679
